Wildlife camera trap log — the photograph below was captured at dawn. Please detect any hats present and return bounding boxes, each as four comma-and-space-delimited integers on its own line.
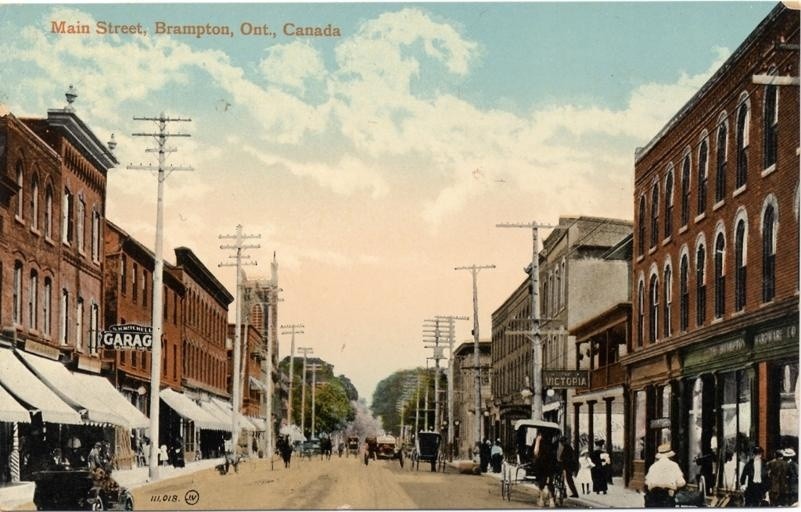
782, 448, 796, 457
580, 449, 588, 455
595, 439, 604, 446
655, 444, 676, 458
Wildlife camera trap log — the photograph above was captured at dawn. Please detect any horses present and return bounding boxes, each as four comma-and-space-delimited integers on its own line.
534, 432, 568, 508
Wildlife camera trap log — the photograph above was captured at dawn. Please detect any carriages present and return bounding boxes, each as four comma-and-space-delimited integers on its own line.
298, 430, 447, 472
33, 458, 134, 511
500, 419, 568, 508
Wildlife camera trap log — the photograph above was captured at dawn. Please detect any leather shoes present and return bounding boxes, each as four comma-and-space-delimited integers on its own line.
583, 490, 607, 495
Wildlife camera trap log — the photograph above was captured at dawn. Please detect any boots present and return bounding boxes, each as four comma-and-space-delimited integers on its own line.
563, 488, 567, 498
569, 485, 578, 497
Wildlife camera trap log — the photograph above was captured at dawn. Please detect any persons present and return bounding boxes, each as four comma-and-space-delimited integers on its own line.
478, 437, 505, 473
557, 431, 612, 499
275, 429, 406, 470
644, 443, 799, 508
46, 428, 242, 509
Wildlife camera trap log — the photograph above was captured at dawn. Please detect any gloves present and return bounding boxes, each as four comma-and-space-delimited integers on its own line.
741, 485, 746, 492
